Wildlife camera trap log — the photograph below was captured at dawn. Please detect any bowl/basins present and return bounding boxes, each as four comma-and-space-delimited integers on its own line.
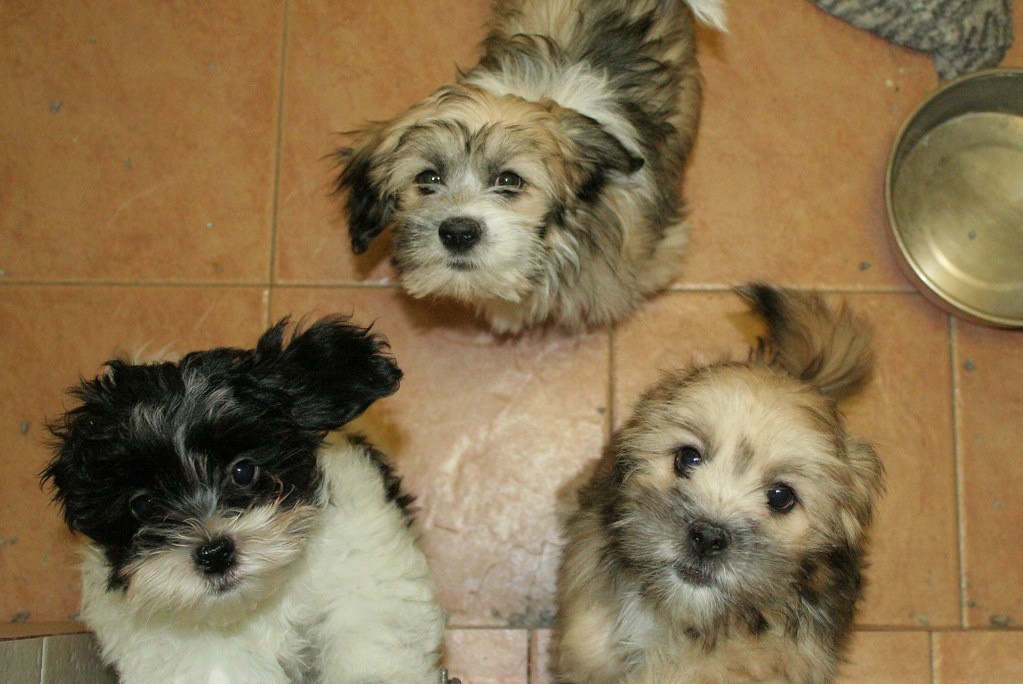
884, 65, 1022, 332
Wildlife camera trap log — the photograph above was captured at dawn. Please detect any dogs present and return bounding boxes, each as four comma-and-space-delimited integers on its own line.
35, 307, 449, 684
544, 282, 888, 684
321, 1, 733, 339
